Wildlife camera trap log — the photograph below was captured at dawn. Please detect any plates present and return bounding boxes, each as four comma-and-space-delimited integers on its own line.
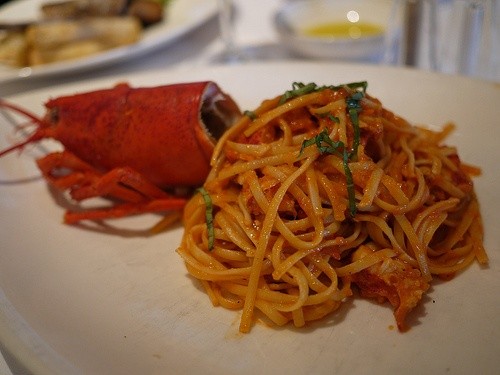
0, 59, 500, 375
271, 0, 399, 63
1, 0, 219, 82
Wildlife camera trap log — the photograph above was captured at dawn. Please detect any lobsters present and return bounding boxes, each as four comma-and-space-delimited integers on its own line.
0, 81, 243, 236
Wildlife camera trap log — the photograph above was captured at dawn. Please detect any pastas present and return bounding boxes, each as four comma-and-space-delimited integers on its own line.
146, 82, 491, 336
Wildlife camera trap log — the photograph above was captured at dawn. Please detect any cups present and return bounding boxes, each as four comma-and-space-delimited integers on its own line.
214, 1, 283, 61
392, 0, 499, 82
